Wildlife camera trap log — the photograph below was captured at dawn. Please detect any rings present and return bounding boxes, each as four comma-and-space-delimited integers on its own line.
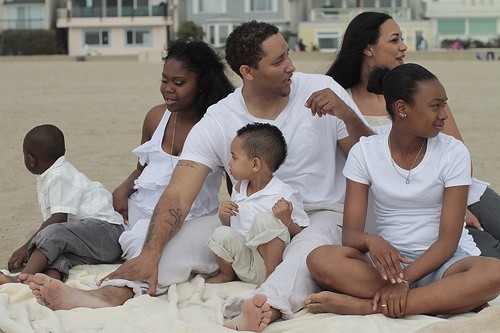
381, 304, 387, 307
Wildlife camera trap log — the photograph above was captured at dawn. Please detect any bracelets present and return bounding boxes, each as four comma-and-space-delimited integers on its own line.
286, 218, 291, 227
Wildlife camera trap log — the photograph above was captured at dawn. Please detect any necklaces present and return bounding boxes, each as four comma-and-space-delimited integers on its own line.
170, 112, 178, 154
388, 134, 426, 184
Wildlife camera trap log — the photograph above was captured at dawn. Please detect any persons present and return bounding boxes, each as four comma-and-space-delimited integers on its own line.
0, 124, 127, 285
113, 38, 236, 233
30, 20, 378, 333
325, 11, 500, 260
205, 122, 311, 285
305, 63, 500, 319
